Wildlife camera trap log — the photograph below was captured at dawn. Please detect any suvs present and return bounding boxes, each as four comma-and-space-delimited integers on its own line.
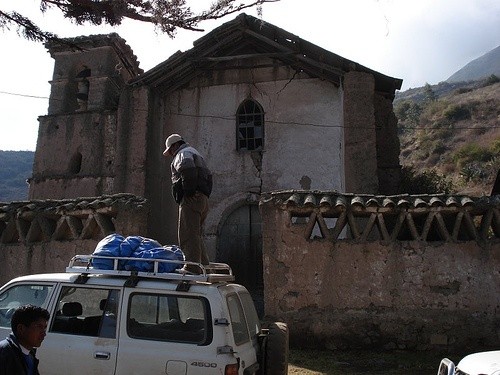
0, 255, 290, 375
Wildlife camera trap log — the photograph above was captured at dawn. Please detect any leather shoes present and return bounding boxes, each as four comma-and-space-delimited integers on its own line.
174, 265, 200, 275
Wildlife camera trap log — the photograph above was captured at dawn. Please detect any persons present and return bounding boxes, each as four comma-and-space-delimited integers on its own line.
162, 134, 216, 277
0, 300, 51, 375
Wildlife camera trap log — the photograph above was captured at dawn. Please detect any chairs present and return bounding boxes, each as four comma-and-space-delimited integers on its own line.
84, 299, 109, 336
53, 302, 85, 335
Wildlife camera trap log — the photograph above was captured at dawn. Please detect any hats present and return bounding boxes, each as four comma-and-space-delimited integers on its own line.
162, 134, 183, 156
